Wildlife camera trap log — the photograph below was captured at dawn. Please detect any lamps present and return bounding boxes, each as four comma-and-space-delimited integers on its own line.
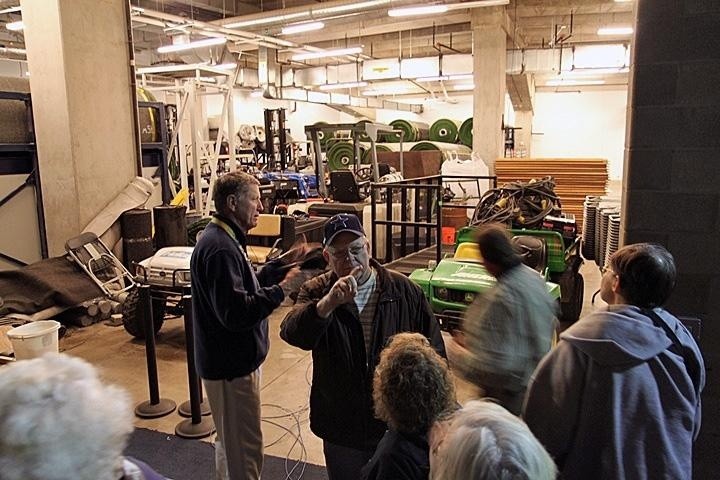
155, 1, 451, 65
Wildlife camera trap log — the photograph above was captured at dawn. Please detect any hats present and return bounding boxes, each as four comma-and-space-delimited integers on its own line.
323, 213, 367, 247
511, 236, 543, 265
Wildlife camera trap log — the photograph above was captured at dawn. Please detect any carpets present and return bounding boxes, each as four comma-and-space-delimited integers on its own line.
121, 425, 331, 480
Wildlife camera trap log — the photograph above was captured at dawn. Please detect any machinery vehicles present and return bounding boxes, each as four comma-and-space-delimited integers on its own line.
304, 118, 406, 222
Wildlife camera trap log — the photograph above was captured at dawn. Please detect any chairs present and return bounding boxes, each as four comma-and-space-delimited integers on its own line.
244, 213, 284, 264
511, 234, 549, 275
451, 242, 485, 262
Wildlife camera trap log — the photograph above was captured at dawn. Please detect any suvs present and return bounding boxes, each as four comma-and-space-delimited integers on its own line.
118, 212, 332, 345
406, 212, 584, 369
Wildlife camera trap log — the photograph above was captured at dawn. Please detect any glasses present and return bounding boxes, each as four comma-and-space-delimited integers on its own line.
328, 242, 366, 258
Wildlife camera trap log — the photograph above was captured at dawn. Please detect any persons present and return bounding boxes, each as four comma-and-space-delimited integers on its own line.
190, 170, 302, 479
360, 333, 458, 480
280, 213, 448, 480
429, 399, 560, 479
522, 242, 706, 479
1, 352, 169, 480
448, 228, 559, 416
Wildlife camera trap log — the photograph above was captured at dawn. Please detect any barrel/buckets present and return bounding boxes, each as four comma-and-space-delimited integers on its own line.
119, 208, 153, 274
441, 226, 455, 244
152, 204, 188, 249
6, 320, 61, 362
440, 201, 467, 243
